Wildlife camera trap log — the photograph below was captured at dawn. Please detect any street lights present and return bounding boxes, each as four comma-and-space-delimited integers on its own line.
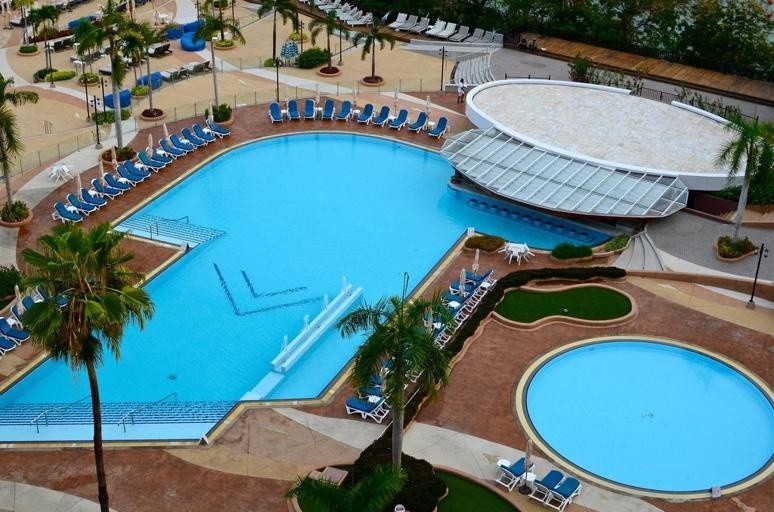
97, 77, 108, 112
90, 95, 100, 143
298, 20, 305, 53
80, 74, 91, 118
439, 45, 448, 90
748, 243, 769, 302
44, 42, 55, 83
272, 57, 283, 102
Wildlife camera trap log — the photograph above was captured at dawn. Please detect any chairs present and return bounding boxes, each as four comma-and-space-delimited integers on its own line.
373, 106, 390, 128
389, 109, 407, 131
0, 272, 96, 360
9, 1, 212, 83
300, 0, 503, 48
269, 103, 284, 124
336, 101, 352, 122
494, 457, 582, 512
52, 117, 231, 226
304, 99, 316, 121
287, 100, 300, 121
408, 112, 427, 134
357, 104, 374, 125
498, 241, 536, 267
428, 116, 450, 142
322, 100, 335, 120
344, 270, 500, 424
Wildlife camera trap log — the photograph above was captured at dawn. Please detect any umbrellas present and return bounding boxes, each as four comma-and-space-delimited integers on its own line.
351, 85, 357, 110
425, 308, 432, 331
313, 79, 321, 110
206, 98, 213, 124
424, 95, 431, 127
470, 248, 479, 277
522, 438, 531, 488
14, 285, 23, 318
75, 122, 172, 202
392, 88, 399, 116
280, 40, 300, 67
457, 267, 465, 296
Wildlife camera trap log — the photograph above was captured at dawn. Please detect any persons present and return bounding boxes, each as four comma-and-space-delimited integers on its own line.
456, 78, 466, 104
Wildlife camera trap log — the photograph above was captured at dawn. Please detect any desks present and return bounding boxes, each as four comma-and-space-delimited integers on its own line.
387, 115, 396, 124
281, 109, 288, 118
315, 106, 323, 117
427, 121, 435, 130
353, 109, 360, 119
